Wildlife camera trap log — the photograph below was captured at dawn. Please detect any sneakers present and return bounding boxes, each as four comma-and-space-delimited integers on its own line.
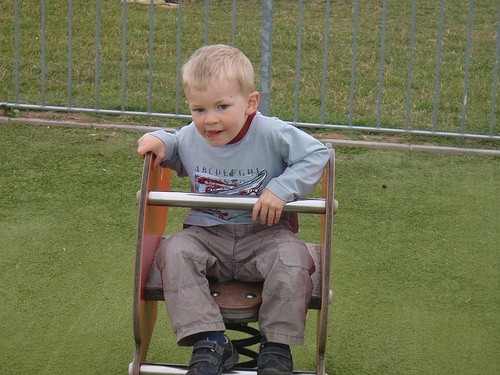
259, 337, 294, 375
185, 334, 241, 375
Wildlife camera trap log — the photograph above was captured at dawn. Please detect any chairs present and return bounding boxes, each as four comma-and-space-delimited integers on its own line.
127, 142, 341, 375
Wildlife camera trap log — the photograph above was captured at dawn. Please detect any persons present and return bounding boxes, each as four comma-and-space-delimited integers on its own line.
137, 44, 330, 375
